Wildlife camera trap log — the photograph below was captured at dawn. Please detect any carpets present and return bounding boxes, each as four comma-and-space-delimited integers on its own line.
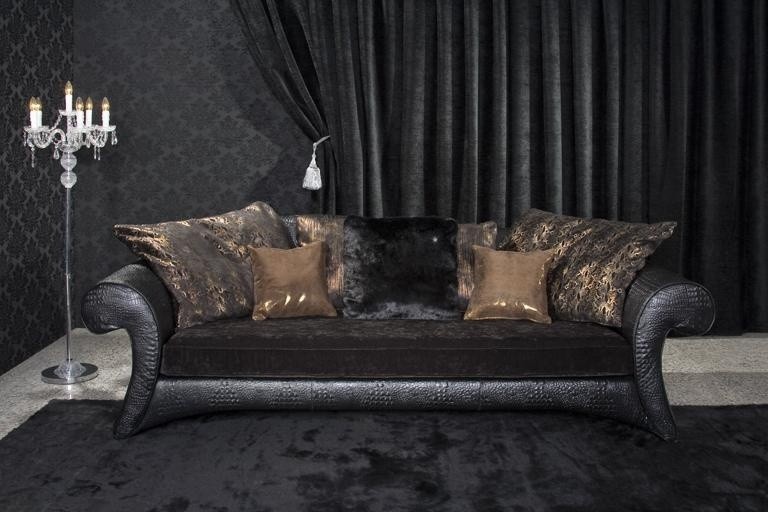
0, 397, 767, 511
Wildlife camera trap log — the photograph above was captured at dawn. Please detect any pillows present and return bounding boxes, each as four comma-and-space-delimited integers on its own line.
245, 241, 337, 321
504, 208, 679, 329
114, 201, 296, 329
295, 215, 498, 313
342, 216, 461, 322
464, 243, 556, 326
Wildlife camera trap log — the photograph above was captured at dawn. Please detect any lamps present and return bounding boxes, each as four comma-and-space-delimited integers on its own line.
21, 79, 118, 386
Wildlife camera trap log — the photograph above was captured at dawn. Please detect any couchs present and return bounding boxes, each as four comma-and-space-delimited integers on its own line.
79, 216, 716, 443
